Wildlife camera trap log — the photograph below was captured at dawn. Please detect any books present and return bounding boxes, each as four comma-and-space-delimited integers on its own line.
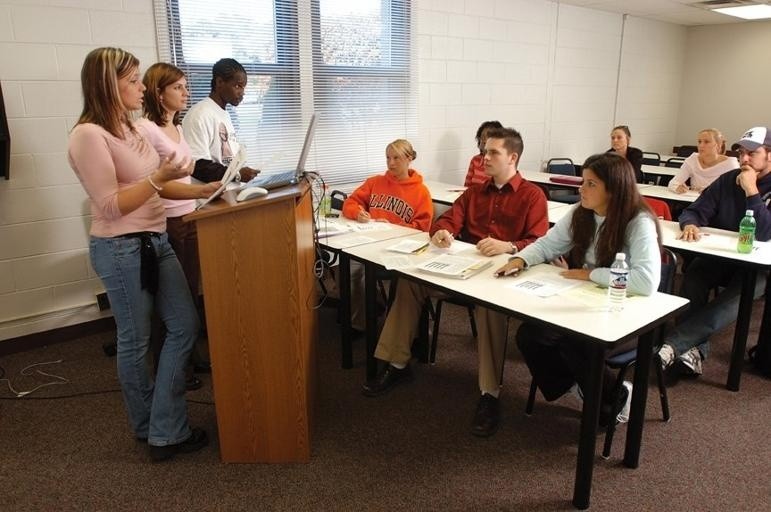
418, 252, 493, 278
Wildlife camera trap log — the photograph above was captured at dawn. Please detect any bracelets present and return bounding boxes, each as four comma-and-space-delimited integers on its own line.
147, 175, 163, 192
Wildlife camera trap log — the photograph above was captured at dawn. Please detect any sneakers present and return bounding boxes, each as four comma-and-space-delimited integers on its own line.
616, 380, 633, 423
657, 343, 674, 370
679, 346, 703, 375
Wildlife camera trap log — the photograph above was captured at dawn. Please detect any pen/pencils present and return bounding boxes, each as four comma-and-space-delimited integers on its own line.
498, 267, 521, 277
698, 232, 710, 236
437, 233, 453, 243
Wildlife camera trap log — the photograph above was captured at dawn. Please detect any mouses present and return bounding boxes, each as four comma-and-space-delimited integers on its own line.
237, 186, 267, 201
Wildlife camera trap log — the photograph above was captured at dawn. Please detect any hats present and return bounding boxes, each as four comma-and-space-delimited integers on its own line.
731, 127, 771, 152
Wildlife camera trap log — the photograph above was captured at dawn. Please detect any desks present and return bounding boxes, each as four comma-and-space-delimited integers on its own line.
339, 231, 692, 511
641, 165, 680, 177
638, 186, 701, 204
315, 204, 423, 372
660, 155, 686, 165
424, 179, 567, 213
183, 169, 320, 464
548, 204, 770, 393
521, 170, 651, 193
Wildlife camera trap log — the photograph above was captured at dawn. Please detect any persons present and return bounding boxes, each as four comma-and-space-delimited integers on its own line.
593, 125, 644, 186
464, 121, 503, 188
132, 63, 209, 391
361, 128, 549, 436
67, 47, 247, 458
492, 153, 663, 431
667, 128, 746, 272
658, 124, 771, 376
182, 58, 261, 208
342, 139, 433, 339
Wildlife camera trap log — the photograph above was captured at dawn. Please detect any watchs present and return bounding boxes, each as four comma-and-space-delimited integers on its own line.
508, 240, 518, 256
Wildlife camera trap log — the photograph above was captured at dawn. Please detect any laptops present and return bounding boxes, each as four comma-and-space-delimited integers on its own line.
236, 113, 320, 194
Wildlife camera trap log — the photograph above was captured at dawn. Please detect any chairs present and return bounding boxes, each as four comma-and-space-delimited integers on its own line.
373, 267, 436, 322
523, 246, 678, 460
641, 151, 660, 185
319, 190, 347, 296
430, 296, 511, 388
659, 158, 690, 187
547, 158, 581, 204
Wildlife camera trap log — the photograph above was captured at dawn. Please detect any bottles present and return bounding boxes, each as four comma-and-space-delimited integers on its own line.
317, 184, 332, 219
607, 253, 629, 313
737, 210, 757, 254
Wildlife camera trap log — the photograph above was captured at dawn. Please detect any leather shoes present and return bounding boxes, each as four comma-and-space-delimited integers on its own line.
471, 394, 501, 437
195, 359, 212, 372
362, 363, 411, 394
185, 375, 202, 390
149, 426, 208, 461
